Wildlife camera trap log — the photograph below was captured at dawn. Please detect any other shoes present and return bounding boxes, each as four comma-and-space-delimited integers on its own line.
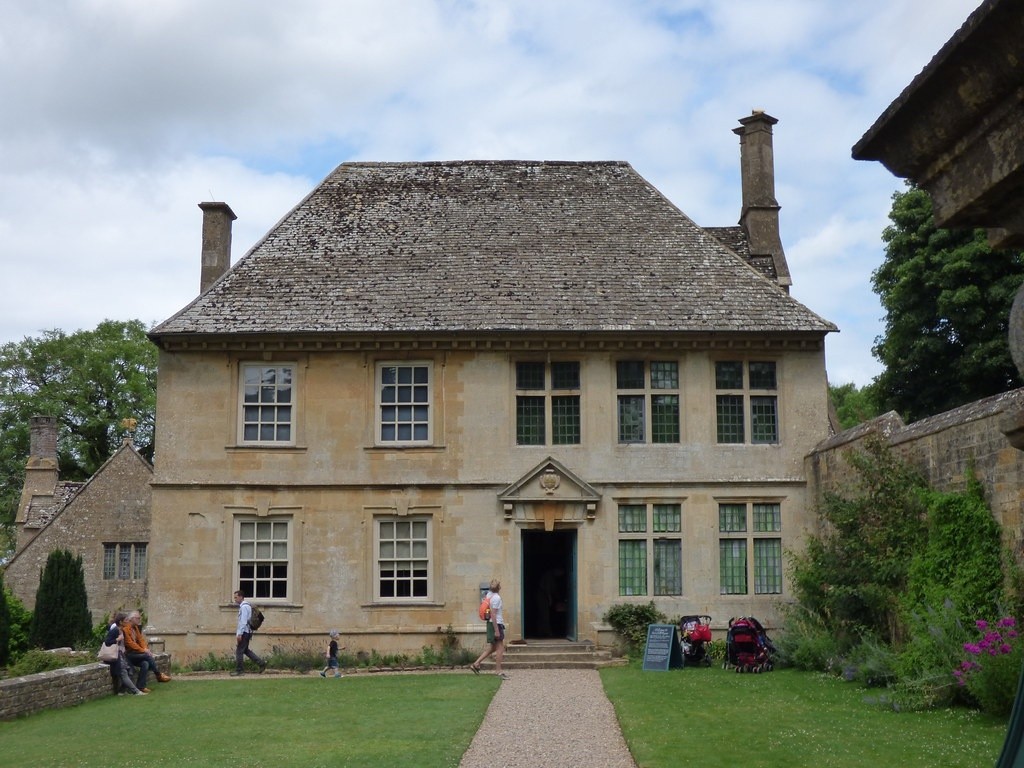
156, 673, 171, 682
135, 691, 148, 695
139, 687, 151, 692
230, 670, 244, 676
470, 664, 481, 674
335, 673, 342, 677
118, 691, 133, 696
497, 672, 510, 680
320, 672, 326, 677
259, 661, 268, 673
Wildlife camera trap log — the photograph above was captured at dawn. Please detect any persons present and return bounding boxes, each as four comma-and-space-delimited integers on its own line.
470, 580, 510, 679
320, 630, 345, 677
231, 590, 268, 675
104, 611, 172, 695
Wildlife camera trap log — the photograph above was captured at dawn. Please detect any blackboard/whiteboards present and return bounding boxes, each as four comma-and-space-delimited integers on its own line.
643, 624, 683, 671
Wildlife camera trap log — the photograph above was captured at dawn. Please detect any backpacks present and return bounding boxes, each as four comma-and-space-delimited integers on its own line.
480, 593, 495, 620
241, 602, 264, 630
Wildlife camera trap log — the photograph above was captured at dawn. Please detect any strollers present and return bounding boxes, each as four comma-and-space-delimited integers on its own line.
723, 616, 774, 674
679, 615, 713, 668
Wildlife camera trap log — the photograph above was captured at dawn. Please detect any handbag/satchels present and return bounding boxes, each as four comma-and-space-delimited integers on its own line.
97, 642, 119, 661
691, 625, 712, 642
